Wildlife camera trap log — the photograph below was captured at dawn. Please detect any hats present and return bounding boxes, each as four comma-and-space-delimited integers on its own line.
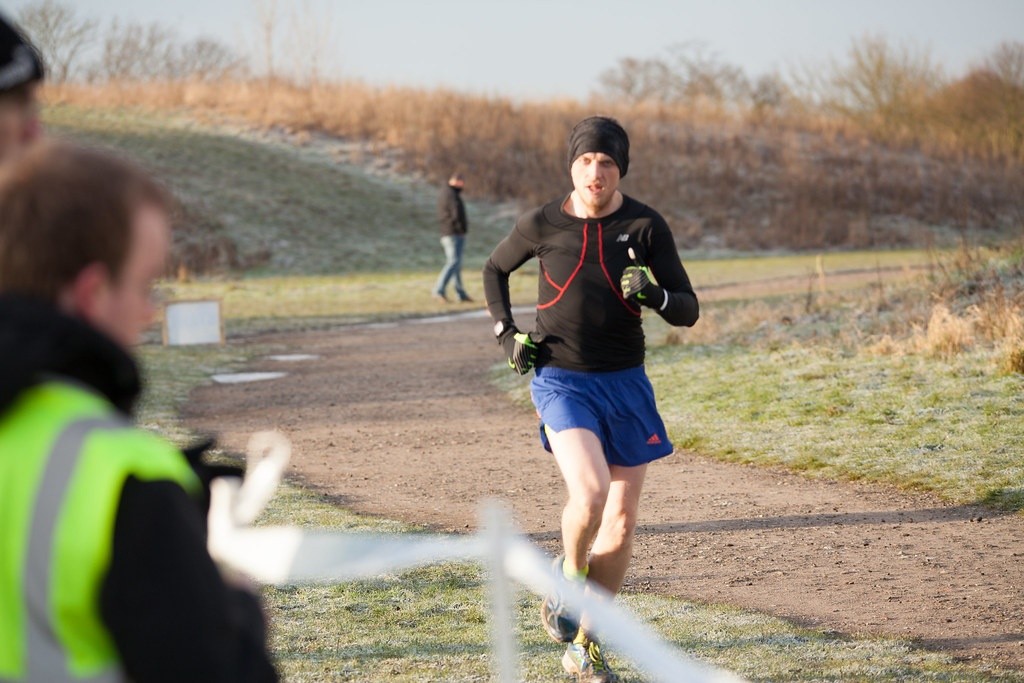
567, 116, 629, 180
0, 19, 44, 95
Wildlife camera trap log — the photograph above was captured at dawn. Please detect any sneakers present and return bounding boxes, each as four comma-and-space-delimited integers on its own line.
561, 642, 621, 683
540, 554, 587, 645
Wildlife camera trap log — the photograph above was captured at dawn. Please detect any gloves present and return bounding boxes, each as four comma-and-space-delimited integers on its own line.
621, 247, 665, 309
496, 325, 547, 375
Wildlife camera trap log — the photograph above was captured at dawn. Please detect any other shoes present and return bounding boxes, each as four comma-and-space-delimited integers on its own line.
431, 291, 447, 303
458, 296, 473, 303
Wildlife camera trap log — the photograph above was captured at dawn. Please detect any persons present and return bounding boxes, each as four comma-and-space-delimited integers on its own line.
0, 17, 47, 166
483, 118, 700, 683
431, 171, 473, 304
0, 145, 281, 683
